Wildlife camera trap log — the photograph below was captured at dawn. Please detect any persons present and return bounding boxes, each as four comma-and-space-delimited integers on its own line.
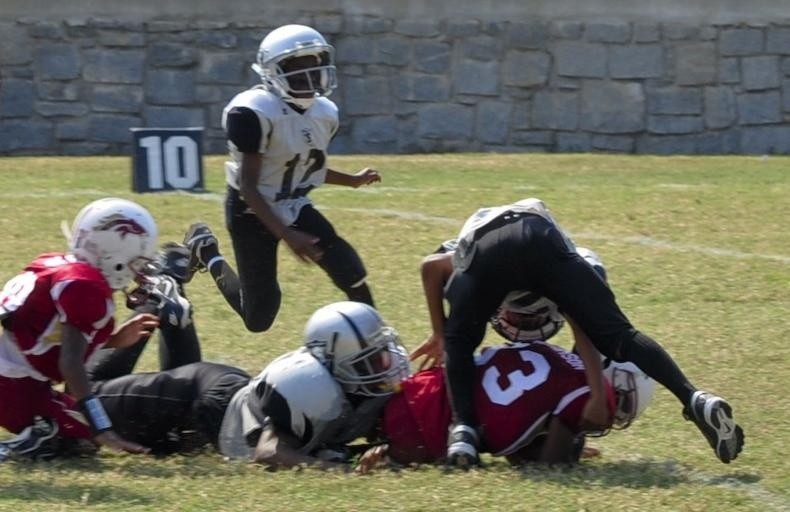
409, 197, 746, 470
65, 240, 410, 477
0, 196, 162, 458
176, 23, 381, 332
373, 341, 652, 468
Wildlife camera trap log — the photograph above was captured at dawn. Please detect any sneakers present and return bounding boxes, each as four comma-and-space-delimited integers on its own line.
157, 237, 204, 282
0, 419, 57, 458
182, 222, 218, 274
447, 422, 479, 466
689, 389, 748, 464
146, 268, 194, 334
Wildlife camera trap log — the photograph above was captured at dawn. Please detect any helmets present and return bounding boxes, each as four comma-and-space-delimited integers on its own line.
250, 23, 340, 111
304, 299, 411, 397
489, 295, 564, 346
66, 197, 161, 291
605, 357, 654, 432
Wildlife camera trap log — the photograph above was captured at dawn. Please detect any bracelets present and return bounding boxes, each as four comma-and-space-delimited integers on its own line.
79, 393, 112, 436
